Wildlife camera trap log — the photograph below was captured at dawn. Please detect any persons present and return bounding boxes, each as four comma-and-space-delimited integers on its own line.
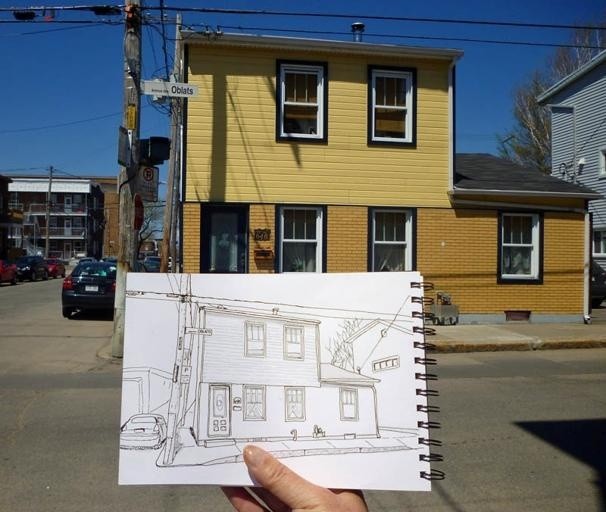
218, 444, 368, 512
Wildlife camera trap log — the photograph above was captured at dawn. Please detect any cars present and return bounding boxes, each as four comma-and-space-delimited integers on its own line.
591, 258, 606, 308
0, 256, 172, 320
